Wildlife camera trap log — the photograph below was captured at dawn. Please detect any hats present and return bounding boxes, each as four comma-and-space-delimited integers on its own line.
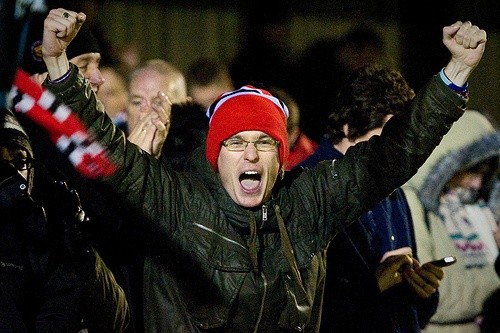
20, 9, 109, 76
0, 107, 34, 159
206, 85, 289, 172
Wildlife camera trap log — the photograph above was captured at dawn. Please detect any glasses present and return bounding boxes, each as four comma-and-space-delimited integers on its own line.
0, 157, 34, 170
223, 135, 280, 152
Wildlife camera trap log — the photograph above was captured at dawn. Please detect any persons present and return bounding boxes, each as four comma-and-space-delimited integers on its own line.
0, 0, 237, 333
228, 24, 499, 333
41, 6, 488, 333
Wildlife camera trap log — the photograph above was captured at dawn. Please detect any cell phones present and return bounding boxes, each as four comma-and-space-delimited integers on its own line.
419, 256, 457, 268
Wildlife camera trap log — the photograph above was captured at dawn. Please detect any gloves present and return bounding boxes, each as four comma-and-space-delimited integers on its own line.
39, 180, 83, 229
